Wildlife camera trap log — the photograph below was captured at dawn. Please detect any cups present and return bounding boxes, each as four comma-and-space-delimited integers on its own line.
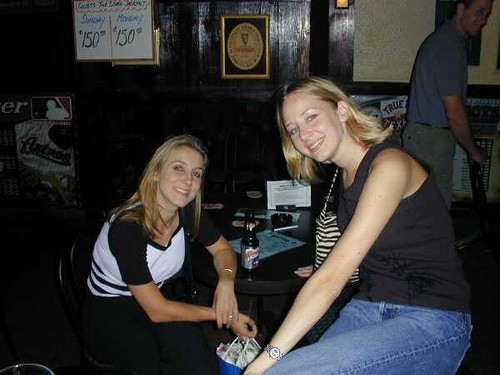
0, 364, 55, 375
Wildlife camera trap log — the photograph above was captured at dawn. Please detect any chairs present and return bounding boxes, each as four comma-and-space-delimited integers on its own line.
58, 234, 145, 375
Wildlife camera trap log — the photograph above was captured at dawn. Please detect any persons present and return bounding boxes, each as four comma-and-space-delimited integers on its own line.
403, 0, 495, 210
244, 76, 475, 375
79, 134, 258, 375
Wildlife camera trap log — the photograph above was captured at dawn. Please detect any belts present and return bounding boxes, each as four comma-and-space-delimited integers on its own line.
416, 123, 451, 130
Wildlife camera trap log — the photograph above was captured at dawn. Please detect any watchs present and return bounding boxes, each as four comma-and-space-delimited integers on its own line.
263, 343, 285, 362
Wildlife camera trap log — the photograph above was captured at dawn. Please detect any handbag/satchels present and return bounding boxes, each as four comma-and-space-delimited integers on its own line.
215, 333, 263, 375
312, 169, 360, 285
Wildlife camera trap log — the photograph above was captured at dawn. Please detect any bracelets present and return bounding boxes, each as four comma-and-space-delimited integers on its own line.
223, 268, 233, 274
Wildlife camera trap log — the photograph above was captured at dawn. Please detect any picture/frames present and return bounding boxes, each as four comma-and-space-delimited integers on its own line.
113, 29, 161, 66
221, 14, 270, 80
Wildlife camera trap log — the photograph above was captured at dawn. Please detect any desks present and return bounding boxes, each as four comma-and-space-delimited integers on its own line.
191, 194, 320, 329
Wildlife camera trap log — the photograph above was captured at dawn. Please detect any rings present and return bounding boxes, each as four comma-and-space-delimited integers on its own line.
228, 315, 233, 319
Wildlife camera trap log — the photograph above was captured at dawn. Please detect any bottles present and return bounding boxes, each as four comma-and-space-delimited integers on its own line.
240, 210, 260, 279
